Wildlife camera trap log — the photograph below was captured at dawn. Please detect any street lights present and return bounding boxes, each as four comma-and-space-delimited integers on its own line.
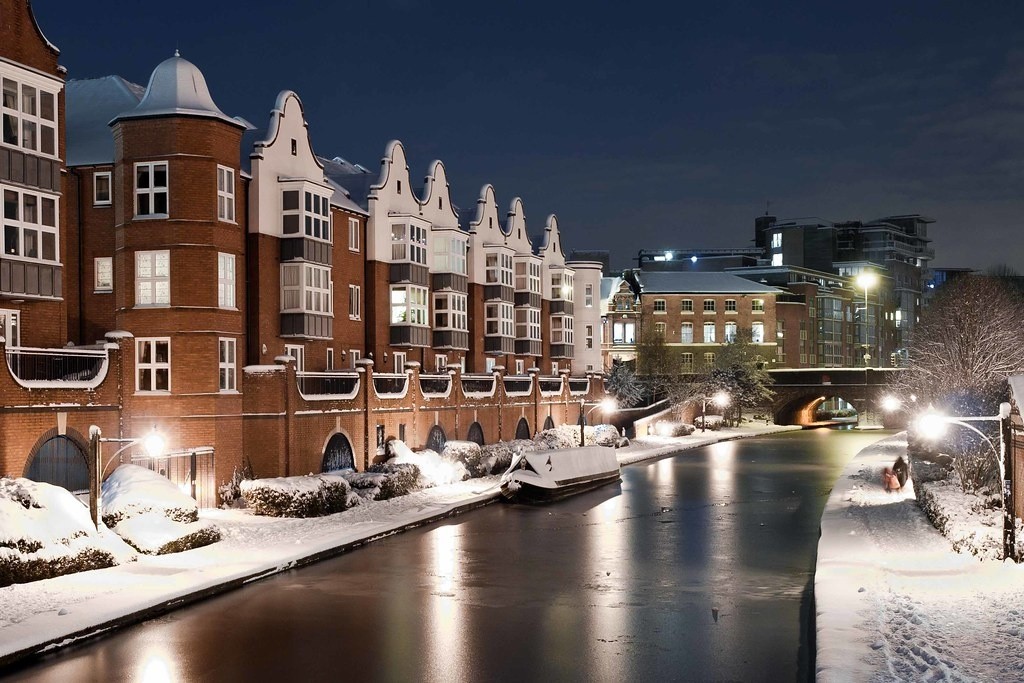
860, 274, 874, 367
580, 402, 614, 446
702, 397, 724, 432
90, 436, 164, 531
885, 398, 912, 420
919, 416, 1015, 561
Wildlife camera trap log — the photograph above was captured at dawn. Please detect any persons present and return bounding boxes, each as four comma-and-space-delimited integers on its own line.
882, 467, 900, 491
893, 455, 908, 488
